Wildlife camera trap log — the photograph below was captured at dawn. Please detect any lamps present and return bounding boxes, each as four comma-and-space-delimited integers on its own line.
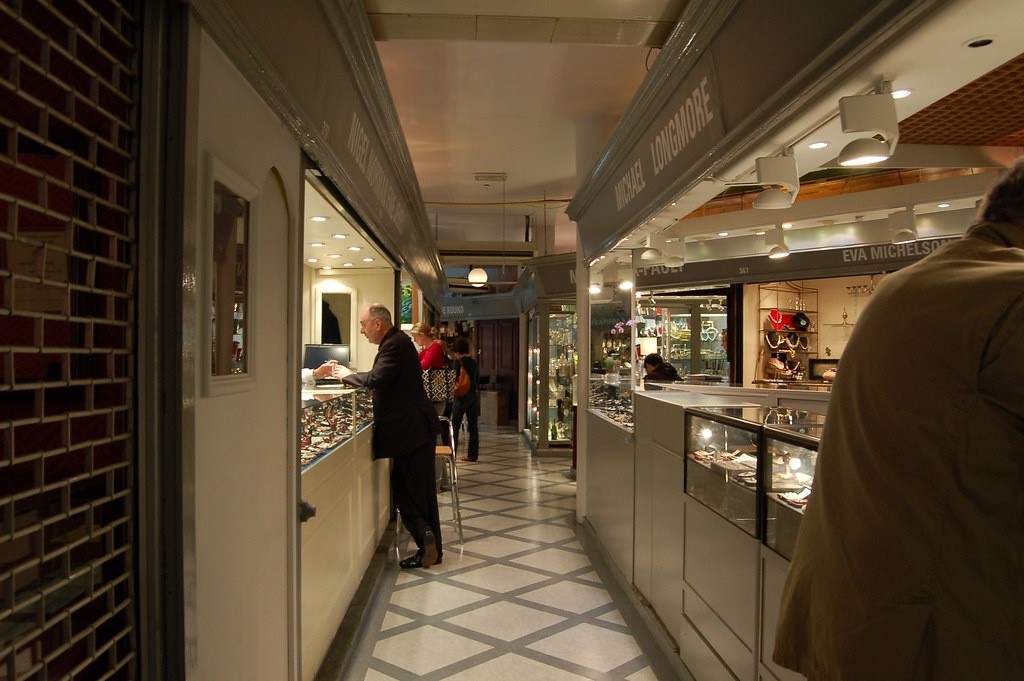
888, 205, 919, 245
468, 263, 488, 287
783, 452, 801, 478
590, 271, 604, 293
765, 223, 790, 259
616, 257, 633, 289
836, 73, 900, 166
641, 230, 687, 267
752, 148, 801, 209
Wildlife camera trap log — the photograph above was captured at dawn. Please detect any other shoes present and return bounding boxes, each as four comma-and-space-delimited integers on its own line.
460, 455, 478, 462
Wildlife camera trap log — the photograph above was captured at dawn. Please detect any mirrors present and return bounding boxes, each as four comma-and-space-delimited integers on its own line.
203, 152, 262, 398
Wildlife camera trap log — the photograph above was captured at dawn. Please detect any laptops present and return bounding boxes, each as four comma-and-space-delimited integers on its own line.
303, 344, 351, 386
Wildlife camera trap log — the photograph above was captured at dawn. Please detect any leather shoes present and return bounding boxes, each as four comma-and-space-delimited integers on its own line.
399, 554, 442, 569
419, 531, 439, 568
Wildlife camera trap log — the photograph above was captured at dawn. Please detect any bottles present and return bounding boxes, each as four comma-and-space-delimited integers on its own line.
551, 418, 557, 440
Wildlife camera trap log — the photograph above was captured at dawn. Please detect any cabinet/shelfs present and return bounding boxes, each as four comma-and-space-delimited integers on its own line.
476, 319, 518, 426
523, 280, 834, 681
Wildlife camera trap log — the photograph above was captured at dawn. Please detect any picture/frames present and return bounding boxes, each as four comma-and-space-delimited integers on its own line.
808, 358, 839, 380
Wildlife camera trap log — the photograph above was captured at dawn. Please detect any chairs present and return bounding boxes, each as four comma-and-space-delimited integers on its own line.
395, 416, 463, 545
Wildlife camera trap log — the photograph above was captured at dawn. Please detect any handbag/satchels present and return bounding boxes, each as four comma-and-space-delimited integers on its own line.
422, 343, 456, 406
454, 359, 471, 396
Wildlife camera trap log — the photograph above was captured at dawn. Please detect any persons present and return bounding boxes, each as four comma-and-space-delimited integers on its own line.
642, 353, 682, 381
721, 329, 727, 351
410, 322, 451, 491
334, 303, 443, 569
771, 156, 1024, 681
302, 360, 338, 384
445, 339, 481, 462
